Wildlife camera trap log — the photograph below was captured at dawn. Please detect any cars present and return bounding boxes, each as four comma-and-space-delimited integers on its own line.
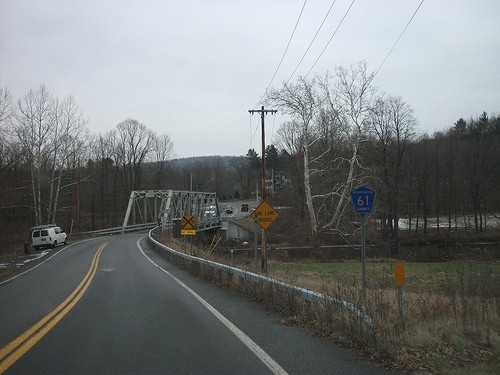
225, 206, 233, 214
241, 203, 248, 212
205, 206, 216, 217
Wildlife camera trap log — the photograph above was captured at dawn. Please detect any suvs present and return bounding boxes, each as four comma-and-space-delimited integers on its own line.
31, 224, 68, 250
